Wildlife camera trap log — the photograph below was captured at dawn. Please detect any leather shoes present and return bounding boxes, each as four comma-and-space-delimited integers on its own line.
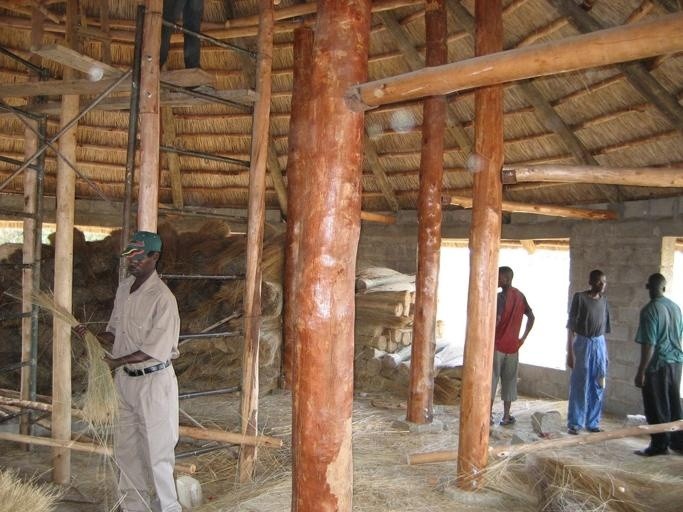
633, 448, 670, 457
669, 439, 683, 451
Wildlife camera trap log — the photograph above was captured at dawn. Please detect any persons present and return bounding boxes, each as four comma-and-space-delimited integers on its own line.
74, 230, 182, 512
491, 266, 536, 426
565, 269, 612, 435
633, 273, 683, 457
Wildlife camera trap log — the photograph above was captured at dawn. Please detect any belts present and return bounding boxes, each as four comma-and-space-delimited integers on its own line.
123, 360, 169, 377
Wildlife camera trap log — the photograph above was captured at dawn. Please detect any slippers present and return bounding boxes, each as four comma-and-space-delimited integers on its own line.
500, 414, 515, 426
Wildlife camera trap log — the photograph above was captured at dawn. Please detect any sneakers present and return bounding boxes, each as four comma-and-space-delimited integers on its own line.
592, 426, 604, 432
568, 427, 578, 434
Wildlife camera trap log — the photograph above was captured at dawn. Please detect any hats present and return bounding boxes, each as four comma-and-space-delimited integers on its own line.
120, 231, 164, 259
